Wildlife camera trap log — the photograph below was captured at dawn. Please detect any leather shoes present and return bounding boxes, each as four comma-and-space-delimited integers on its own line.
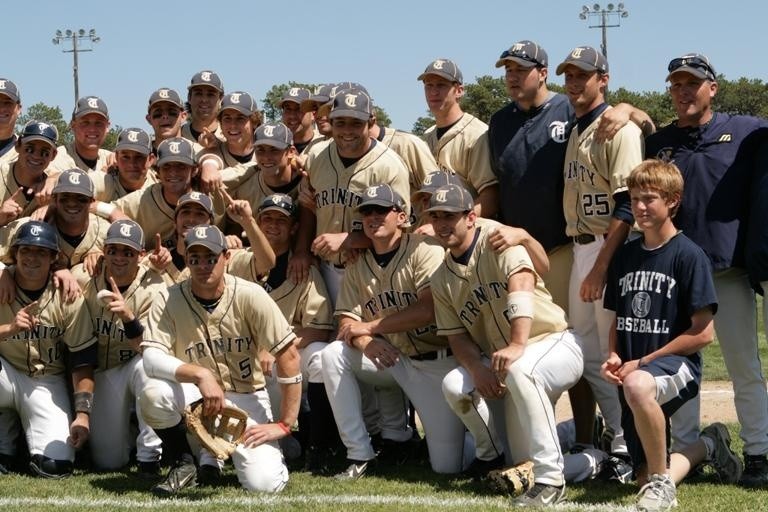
406, 347, 457, 359
570, 229, 613, 246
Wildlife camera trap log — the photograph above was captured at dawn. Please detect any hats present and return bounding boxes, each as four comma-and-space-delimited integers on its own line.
116, 127, 197, 167
354, 184, 407, 211
556, 45, 609, 75
250, 119, 293, 151
52, 168, 95, 198
277, 82, 373, 120
11, 221, 60, 251
417, 59, 464, 84
665, 53, 717, 81
257, 193, 298, 220
149, 70, 257, 116
173, 191, 228, 255
23, 119, 58, 149
72, 96, 109, 120
0, 78, 20, 104
495, 40, 548, 69
414, 170, 475, 214
103, 219, 145, 252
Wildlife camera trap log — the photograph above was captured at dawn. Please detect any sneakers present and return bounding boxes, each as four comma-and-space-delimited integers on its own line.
138, 456, 199, 496
699, 421, 768, 488
335, 452, 377, 482
509, 481, 568, 512
635, 472, 679, 512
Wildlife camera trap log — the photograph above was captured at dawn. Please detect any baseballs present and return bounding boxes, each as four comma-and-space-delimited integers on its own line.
96, 289, 115, 309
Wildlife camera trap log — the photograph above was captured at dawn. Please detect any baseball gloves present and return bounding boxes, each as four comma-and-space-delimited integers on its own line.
488, 460, 535, 493
187, 402, 247, 460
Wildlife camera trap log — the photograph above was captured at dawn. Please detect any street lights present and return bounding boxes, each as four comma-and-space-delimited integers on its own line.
51, 23, 102, 109
580, 2, 629, 102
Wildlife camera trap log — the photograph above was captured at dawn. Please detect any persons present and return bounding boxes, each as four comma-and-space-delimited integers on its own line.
744, 123, 768, 383
600, 157, 745, 509
550, 44, 649, 484
486, 39, 660, 459
483, 406, 616, 498
424, 182, 587, 508
639, 54, 767, 486
0, 56, 501, 493
